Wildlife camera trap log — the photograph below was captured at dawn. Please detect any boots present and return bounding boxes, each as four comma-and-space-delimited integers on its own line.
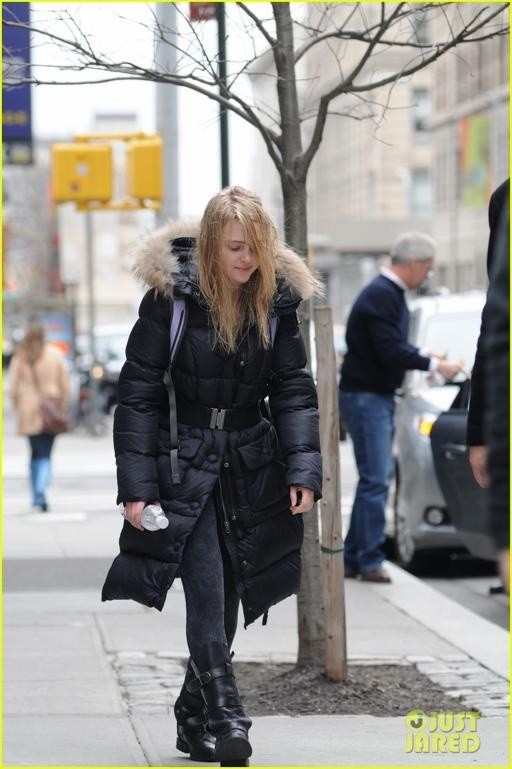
189, 640, 253, 766
172, 655, 218, 763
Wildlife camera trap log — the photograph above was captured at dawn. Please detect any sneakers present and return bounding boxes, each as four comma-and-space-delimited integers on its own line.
344, 560, 393, 585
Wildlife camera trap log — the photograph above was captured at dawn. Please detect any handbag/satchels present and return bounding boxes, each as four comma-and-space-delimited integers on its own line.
37, 392, 74, 435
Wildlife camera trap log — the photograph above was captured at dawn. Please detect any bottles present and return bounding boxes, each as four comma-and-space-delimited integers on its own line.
118, 497, 170, 533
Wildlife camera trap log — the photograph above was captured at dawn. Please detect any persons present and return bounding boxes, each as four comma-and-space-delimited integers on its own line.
99, 187, 325, 768
468, 183, 509, 490
336, 230, 470, 582
4, 321, 70, 511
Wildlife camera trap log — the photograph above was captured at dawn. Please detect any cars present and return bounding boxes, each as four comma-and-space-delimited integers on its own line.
307, 293, 510, 571
75, 325, 137, 414
